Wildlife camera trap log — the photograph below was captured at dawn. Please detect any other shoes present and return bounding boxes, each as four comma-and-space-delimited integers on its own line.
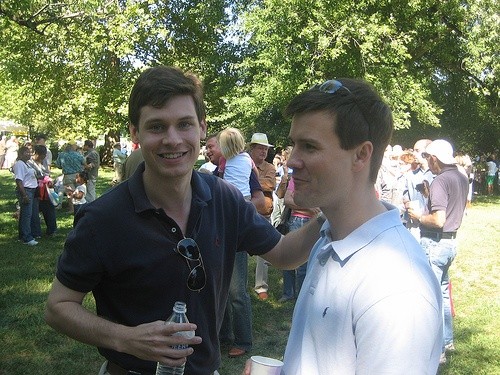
439, 343, 455, 364
277, 295, 296, 304
24, 240, 39, 246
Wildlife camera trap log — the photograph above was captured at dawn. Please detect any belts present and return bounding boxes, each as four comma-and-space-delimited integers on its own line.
420, 231, 456, 239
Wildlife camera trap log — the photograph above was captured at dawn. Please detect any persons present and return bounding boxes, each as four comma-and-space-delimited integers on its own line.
36, 137, 53, 173
0, 133, 33, 168
195, 117, 499, 362
14, 146, 42, 246
111, 142, 127, 184
19, 146, 59, 239
123, 145, 146, 183
57, 139, 92, 197
42, 65, 381, 375
66, 169, 88, 221
239, 76, 446, 375
80, 139, 99, 203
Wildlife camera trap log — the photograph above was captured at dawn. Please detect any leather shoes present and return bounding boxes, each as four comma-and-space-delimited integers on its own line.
259, 292, 268, 301
228, 346, 246, 357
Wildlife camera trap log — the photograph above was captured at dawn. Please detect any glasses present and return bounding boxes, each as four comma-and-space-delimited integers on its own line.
173, 236, 206, 292
308, 79, 372, 141
36, 138, 42, 140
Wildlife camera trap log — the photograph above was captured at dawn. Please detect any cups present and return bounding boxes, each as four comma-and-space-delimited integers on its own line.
409, 199, 420, 213
250, 356, 284, 375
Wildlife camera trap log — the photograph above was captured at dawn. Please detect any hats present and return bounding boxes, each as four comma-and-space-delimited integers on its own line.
33, 133, 46, 139
68, 140, 77, 145
250, 133, 274, 147
426, 140, 459, 164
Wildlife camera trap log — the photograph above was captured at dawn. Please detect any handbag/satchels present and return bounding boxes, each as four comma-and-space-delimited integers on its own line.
274, 206, 291, 236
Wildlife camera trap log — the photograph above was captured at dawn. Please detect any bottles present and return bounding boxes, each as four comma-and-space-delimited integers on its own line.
67, 186, 73, 199
156, 301, 189, 375
